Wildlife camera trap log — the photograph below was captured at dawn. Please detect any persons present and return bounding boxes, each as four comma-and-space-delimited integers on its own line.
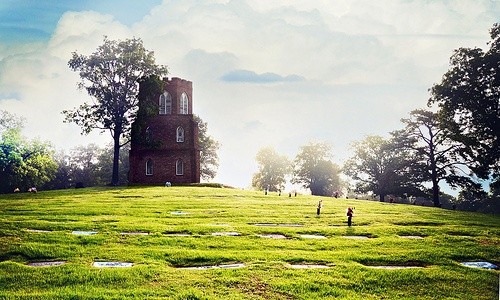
346, 207, 354, 226
316, 200, 322, 215
264, 185, 298, 198
334, 190, 457, 210
